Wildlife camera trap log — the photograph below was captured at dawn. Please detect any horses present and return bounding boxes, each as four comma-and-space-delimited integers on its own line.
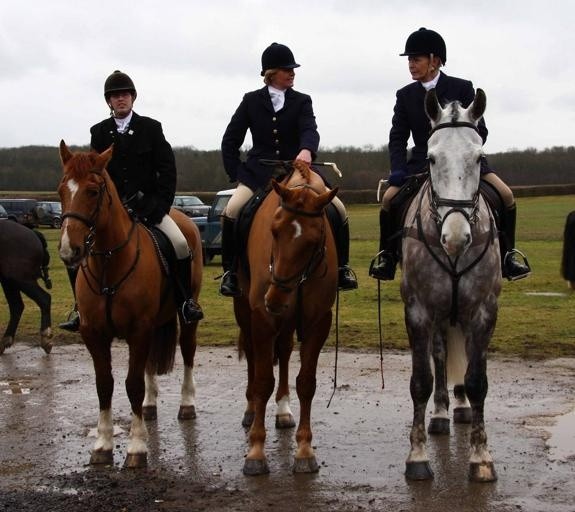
232, 159, 340, 477
0, 218, 54, 356
399, 86, 504, 484
56, 139, 205, 471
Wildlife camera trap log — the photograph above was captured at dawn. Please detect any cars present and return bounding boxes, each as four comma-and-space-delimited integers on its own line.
0, 205, 8, 220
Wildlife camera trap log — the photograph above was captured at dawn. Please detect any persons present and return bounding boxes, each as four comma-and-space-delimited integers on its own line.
56, 72, 204, 331
218, 41, 358, 292
371, 27, 531, 279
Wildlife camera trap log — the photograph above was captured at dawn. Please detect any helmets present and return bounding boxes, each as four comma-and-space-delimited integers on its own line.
399, 28, 446, 63
261, 43, 300, 74
104, 70, 137, 105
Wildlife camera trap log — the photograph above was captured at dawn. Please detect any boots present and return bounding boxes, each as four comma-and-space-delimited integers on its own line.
499, 202, 529, 276
371, 208, 402, 277
57, 267, 80, 331
335, 217, 358, 289
221, 215, 237, 294
174, 255, 203, 322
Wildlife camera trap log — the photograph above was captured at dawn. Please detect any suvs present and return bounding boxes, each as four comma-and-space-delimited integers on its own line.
172, 195, 212, 217
36, 200, 64, 229
0, 198, 45, 229
188, 186, 238, 265
